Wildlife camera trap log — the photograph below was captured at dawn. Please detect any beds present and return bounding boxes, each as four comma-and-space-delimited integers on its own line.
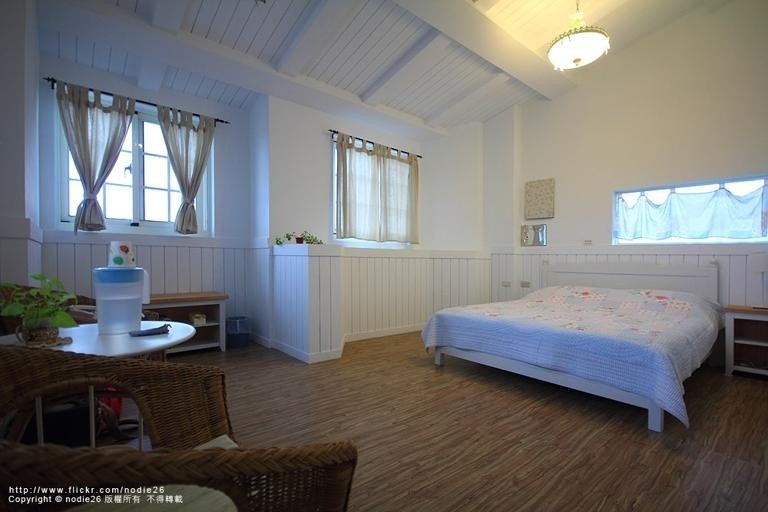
433, 260, 719, 434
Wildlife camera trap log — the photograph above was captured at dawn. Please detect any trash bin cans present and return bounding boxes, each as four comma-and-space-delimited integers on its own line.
225, 316, 249, 350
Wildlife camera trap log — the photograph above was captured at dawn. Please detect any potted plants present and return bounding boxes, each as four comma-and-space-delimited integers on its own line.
272, 230, 324, 248
0, 274, 79, 345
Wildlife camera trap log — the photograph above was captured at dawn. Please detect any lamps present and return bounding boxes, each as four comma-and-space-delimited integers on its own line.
545, 1, 612, 71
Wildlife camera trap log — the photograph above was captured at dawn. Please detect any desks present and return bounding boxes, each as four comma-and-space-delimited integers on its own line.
0, 322, 196, 358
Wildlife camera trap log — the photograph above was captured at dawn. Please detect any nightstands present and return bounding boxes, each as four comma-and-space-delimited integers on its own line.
723, 305, 767, 378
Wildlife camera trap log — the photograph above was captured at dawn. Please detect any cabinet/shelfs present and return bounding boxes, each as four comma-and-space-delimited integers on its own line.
139, 299, 230, 354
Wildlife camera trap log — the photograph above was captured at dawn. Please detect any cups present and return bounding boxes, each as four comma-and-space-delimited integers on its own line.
92, 265, 151, 338
107, 241, 137, 269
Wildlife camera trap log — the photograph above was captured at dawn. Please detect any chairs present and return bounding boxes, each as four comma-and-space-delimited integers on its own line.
0, 343, 357, 512
